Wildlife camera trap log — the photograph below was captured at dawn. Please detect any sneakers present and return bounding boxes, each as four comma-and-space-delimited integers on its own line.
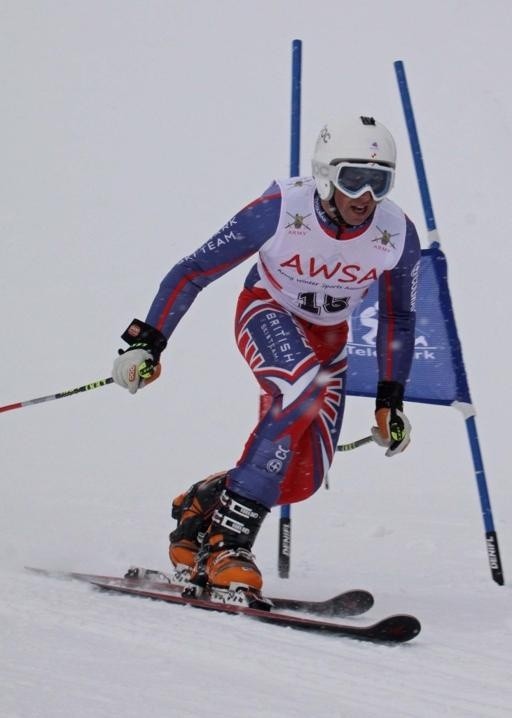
168, 488, 208, 577
201, 524, 261, 589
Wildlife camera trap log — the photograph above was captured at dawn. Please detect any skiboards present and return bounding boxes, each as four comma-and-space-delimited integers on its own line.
20, 558, 421, 643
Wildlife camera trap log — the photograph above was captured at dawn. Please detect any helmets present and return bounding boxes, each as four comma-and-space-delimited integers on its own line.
309, 119, 396, 201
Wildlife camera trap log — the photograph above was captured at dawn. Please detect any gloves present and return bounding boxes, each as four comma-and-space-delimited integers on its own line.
373, 382, 411, 457
112, 319, 168, 393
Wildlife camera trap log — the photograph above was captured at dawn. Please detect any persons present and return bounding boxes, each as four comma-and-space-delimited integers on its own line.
111, 115, 422, 591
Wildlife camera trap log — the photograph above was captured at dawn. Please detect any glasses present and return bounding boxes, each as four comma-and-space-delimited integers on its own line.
311, 161, 394, 202
